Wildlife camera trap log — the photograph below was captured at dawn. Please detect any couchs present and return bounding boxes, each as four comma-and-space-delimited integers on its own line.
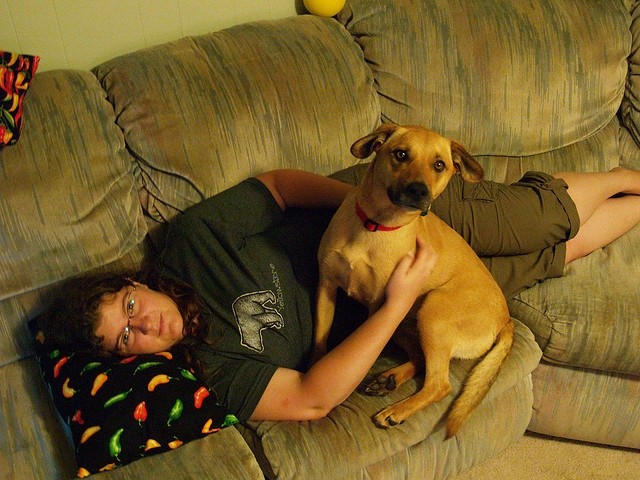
0, 0, 640, 480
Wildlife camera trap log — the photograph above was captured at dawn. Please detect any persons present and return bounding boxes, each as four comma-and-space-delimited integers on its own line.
71, 161, 640, 420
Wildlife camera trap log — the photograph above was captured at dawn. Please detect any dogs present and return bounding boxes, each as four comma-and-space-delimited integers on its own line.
306, 124, 516, 442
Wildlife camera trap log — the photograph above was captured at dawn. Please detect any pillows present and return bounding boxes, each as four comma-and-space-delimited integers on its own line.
0, 51, 41, 145
24, 316, 240, 476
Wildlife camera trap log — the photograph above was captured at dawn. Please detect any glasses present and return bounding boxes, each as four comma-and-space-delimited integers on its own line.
110, 280, 143, 354
